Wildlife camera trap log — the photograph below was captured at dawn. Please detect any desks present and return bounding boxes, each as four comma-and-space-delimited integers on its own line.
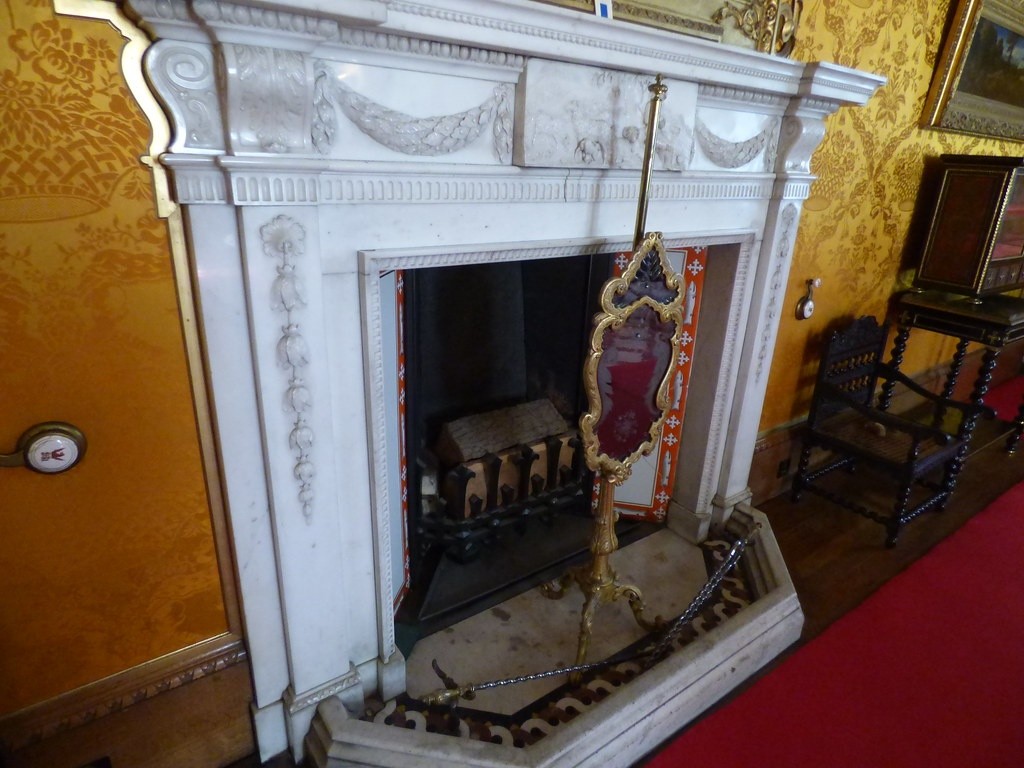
878, 289, 1024, 511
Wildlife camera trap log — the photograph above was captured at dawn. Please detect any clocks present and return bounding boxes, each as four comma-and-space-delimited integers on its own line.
795, 278, 814, 320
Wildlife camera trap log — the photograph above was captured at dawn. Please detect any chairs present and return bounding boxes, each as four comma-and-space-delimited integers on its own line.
792, 315, 998, 546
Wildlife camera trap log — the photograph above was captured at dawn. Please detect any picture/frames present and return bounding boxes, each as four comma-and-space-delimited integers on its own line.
919, 0, 1024, 143
